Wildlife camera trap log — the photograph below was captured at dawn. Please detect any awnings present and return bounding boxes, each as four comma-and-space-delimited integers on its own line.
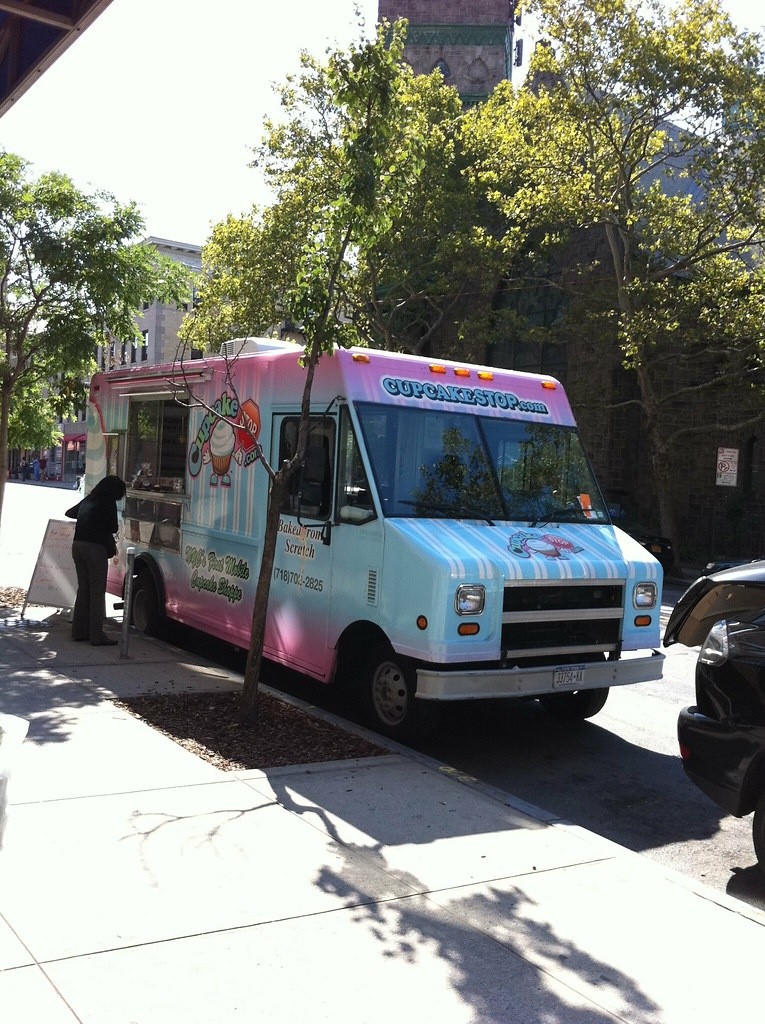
59, 434, 87, 442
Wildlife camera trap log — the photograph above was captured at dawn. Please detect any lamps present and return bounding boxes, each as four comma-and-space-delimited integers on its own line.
28, 466, 35, 480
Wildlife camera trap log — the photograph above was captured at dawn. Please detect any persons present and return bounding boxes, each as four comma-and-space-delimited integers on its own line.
15, 454, 47, 483
65, 475, 127, 647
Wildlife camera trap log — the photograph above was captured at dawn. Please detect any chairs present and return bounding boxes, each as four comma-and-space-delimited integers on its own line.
303, 434, 331, 475
420, 456, 467, 504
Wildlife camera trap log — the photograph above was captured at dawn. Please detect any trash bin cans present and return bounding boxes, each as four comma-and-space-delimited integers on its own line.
76, 476, 81, 490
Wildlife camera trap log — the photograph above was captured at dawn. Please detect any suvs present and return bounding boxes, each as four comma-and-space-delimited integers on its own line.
664, 553, 765, 863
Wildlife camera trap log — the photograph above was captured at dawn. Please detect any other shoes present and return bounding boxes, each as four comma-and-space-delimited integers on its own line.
73, 633, 90, 641
91, 637, 118, 646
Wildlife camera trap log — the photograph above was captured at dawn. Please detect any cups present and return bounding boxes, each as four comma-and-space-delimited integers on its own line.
173, 478, 183, 494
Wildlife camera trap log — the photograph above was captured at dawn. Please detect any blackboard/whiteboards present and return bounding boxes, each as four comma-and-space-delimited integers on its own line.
27, 519, 78, 609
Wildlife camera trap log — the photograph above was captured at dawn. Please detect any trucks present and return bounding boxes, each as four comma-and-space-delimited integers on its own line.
83, 335, 667, 742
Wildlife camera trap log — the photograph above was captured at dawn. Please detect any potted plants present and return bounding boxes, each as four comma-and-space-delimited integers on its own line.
6, 466, 24, 480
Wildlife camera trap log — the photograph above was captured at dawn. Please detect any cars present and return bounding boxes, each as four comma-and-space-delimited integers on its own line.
612, 514, 671, 571
